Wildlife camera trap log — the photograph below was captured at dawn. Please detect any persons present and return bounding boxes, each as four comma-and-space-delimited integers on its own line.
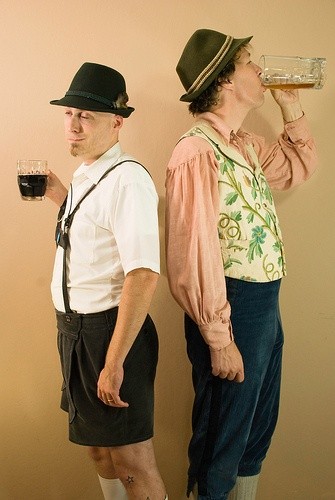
22, 63, 173, 500
166, 30, 318, 500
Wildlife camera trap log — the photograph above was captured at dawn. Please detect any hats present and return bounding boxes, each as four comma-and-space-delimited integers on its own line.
176, 29, 253, 102
50, 61, 136, 118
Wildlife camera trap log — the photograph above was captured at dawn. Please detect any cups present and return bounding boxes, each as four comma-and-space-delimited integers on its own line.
259, 55, 327, 90
17, 159, 48, 201
